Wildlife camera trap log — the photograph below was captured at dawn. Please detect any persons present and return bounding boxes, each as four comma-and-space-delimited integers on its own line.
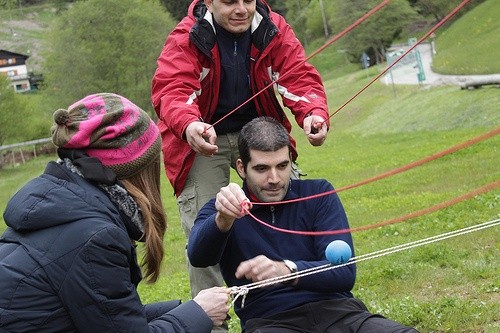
1, 93, 230, 333
27, 68, 38, 91
152, 0, 330, 332
360, 52, 371, 69
187, 117, 421, 333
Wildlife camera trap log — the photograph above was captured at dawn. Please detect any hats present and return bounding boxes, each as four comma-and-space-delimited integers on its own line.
50, 92, 162, 181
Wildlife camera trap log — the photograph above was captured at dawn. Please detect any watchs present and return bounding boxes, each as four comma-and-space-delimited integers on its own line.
282, 257, 297, 285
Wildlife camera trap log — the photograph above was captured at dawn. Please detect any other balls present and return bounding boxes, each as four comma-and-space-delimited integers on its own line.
324, 239, 353, 265
